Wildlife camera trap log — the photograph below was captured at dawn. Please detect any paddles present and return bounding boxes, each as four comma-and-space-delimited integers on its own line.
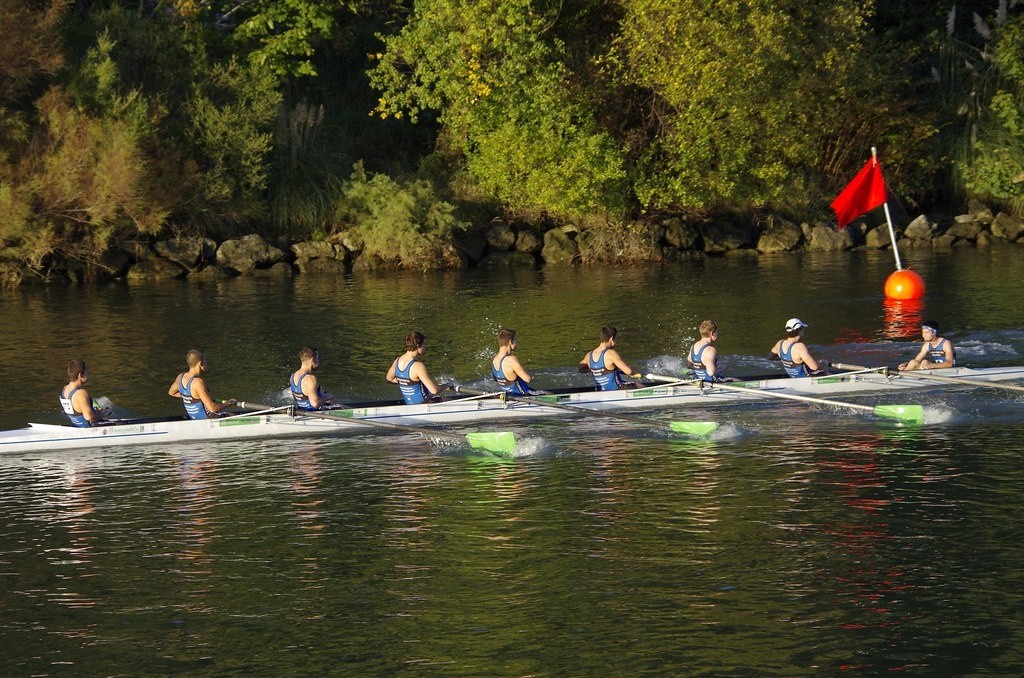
827, 362, 1024, 391
447, 383, 720, 437
630, 373, 923, 421
212, 397, 517, 454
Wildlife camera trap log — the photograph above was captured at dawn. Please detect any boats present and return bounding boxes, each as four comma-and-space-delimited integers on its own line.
0, 367, 1022, 457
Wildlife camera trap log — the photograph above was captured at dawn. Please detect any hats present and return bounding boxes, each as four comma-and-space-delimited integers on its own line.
785, 318, 808, 332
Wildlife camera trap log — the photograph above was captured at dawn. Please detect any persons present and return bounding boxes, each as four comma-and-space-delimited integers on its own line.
577, 325, 647, 391
289, 346, 342, 413
687, 319, 743, 383
61, 358, 115, 428
897, 321, 957, 371
385, 332, 460, 405
167, 349, 237, 420
490, 328, 554, 397
768, 317, 833, 378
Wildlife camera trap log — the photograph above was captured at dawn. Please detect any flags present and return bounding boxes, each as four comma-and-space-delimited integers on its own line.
829, 153, 888, 231
